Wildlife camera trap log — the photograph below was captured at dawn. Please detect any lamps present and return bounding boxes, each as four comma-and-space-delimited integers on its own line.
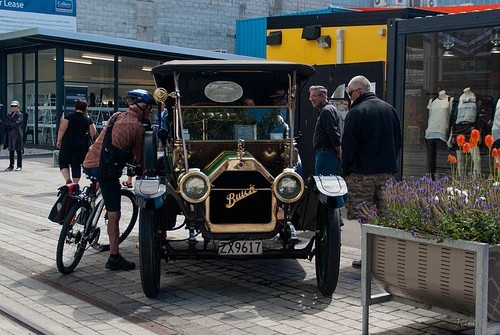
443, 30, 455, 56
488, 26, 500, 53
317, 35, 332, 48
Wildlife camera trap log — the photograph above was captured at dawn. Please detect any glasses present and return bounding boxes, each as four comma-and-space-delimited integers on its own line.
11, 105, 16, 107
309, 93, 322, 100
349, 90, 356, 96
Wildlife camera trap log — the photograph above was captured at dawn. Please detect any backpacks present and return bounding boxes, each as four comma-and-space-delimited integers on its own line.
48, 182, 80, 225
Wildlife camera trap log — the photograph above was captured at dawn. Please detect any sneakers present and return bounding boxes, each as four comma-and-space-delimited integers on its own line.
86, 186, 98, 198
105, 254, 136, 270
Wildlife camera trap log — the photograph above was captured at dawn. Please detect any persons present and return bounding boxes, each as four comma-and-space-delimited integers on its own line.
342, 75, 402, 268
309, 85, 345, 226
5, 100, 24, 171
76, 88, 155, 271
153, 102, 168, 149
56, 99, 96, 182
425, 86, 482, 179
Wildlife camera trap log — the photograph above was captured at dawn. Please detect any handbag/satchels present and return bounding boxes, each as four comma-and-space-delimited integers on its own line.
86, 115, 95, 147
98, 144, 128, 178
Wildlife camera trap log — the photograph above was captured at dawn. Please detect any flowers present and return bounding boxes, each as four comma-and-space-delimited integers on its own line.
354, 129, 500, 245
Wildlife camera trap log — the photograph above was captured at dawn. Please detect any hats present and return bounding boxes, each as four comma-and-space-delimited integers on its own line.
10, 101, 20, 108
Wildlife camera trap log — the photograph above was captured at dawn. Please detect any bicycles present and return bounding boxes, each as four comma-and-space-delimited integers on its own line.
56, 173, 139, 275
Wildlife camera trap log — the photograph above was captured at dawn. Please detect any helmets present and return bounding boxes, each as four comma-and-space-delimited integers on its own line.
127, 89, 157, 106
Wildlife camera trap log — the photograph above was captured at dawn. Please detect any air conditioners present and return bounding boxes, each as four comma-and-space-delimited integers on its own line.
425, 0, 438, 7
394, 0, 407, 5
374, 0, 387, 7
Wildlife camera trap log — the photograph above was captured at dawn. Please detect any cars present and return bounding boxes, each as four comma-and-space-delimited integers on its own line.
135, 60, 349, 299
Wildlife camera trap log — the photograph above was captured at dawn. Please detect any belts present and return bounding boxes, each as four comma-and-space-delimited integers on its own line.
315, 147, 327, 152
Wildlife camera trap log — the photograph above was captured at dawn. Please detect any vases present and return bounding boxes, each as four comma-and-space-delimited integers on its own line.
356, 224, 500, 335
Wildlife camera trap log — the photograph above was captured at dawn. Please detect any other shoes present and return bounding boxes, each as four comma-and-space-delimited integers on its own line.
352, 260, 361, 269
65, 180, 72, 184
5, 165, 23, 171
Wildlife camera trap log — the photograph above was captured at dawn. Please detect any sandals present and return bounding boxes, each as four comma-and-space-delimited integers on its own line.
122, 182, 133, 188
